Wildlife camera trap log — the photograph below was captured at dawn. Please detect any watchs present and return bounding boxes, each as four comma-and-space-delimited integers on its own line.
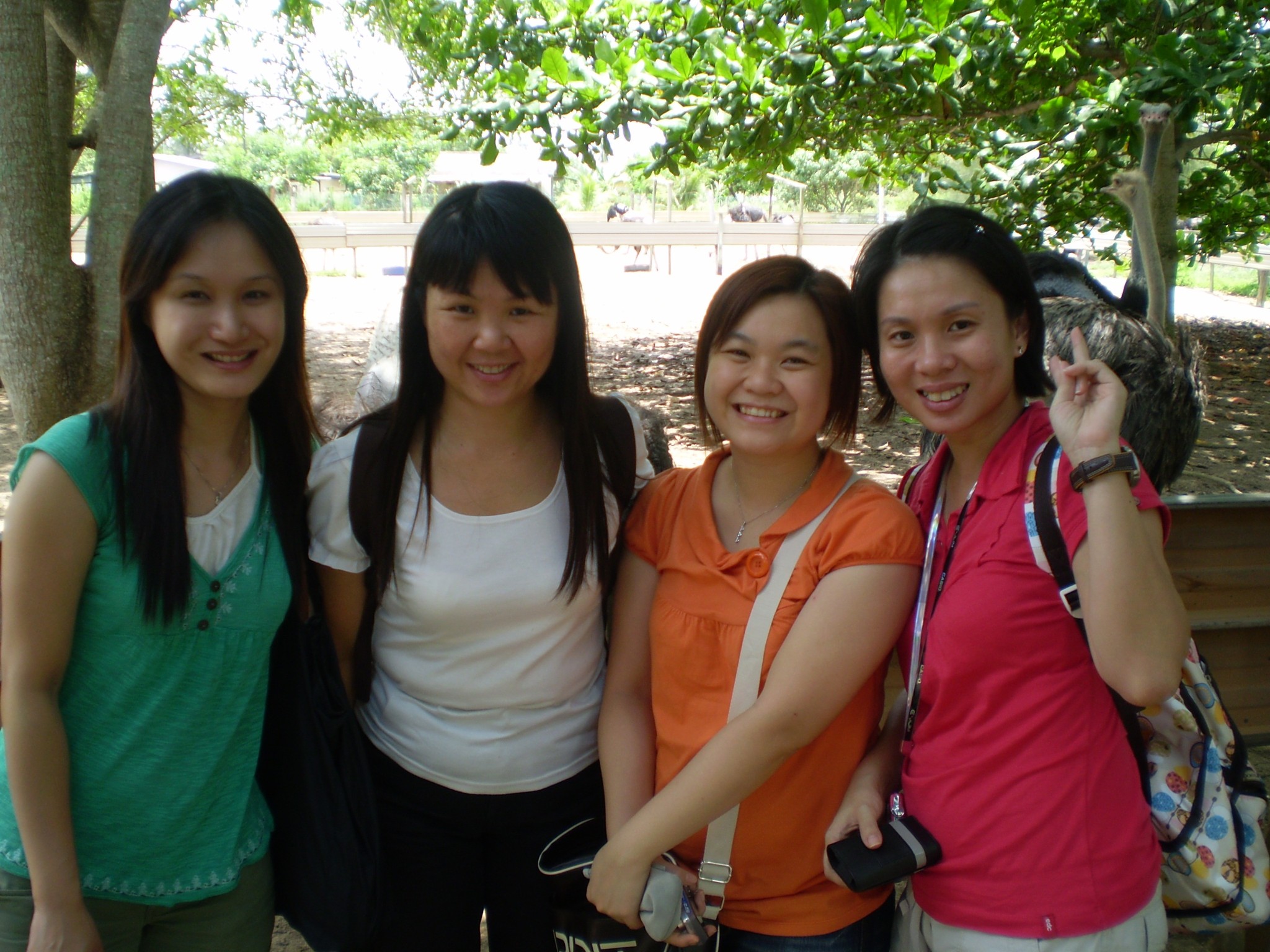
1069, 446, 1141, 493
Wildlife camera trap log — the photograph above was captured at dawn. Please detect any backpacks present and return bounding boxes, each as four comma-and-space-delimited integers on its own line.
904, 442, 1270, 937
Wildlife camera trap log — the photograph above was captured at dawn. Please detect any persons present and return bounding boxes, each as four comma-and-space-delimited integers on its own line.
307, 182, 655, 952
822, 205, 1192, 952
1, 172, 331, 952
585, 255, 926, 952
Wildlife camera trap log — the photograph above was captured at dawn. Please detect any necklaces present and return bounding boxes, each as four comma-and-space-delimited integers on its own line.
182, 429, 248, 505
731, 457, 821, 544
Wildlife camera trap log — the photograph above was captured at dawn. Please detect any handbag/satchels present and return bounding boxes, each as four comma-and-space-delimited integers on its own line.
258, 518, 385, 951
826, 814, 942, 893
534, 810, 726, 952
